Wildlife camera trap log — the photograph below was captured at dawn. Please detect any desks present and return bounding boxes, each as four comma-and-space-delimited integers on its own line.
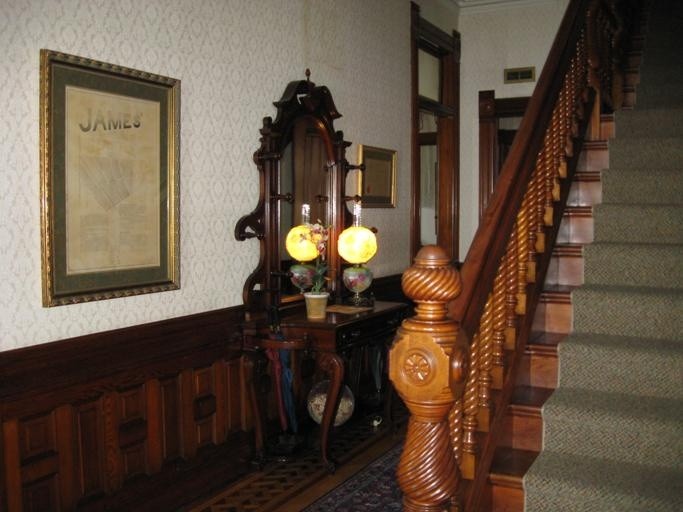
236, 300, 411, 475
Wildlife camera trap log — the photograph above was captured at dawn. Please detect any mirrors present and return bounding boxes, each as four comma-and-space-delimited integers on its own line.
234, 68, 377, 316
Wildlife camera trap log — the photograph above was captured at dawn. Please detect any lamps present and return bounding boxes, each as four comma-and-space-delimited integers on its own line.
337, 204, 378, 307
285, 203, 322, 294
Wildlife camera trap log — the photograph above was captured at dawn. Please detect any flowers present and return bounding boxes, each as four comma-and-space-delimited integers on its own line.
298, 218, 336, 294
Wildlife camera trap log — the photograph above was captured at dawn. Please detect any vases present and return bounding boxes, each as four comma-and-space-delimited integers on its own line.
303, 291, 329, 321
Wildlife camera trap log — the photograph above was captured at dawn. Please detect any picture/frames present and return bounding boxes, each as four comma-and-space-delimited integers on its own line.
357, 143, 398, 209
39, 47, 181, 308
